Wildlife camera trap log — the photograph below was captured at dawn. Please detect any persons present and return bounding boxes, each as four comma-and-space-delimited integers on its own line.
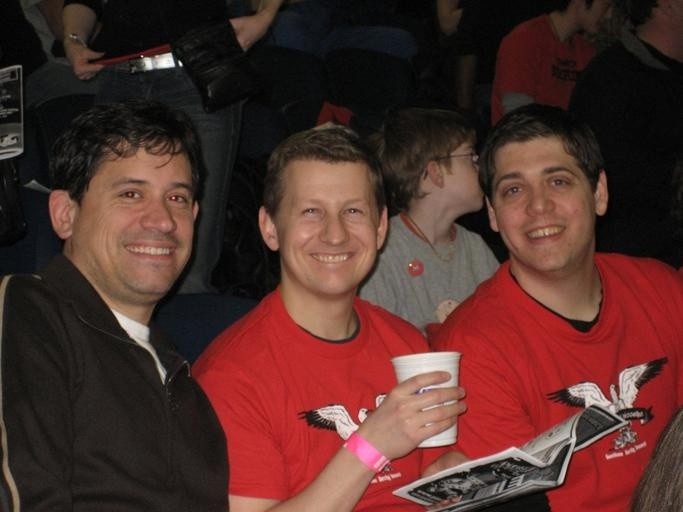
0, 96, 230, 511
188, 127, 481, 512
431, 103, 682, 512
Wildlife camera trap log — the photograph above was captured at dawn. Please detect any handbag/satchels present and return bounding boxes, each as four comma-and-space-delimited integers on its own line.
171, 21, 261, 114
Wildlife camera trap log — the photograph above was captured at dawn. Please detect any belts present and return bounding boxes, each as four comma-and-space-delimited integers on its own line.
106, 51, 185, 74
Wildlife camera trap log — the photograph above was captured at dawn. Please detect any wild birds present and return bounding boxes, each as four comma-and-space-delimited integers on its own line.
546, 356, 669, 454
294, 394, 386, 443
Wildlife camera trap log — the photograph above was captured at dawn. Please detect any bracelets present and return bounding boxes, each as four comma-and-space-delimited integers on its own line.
342, 432, 389, 473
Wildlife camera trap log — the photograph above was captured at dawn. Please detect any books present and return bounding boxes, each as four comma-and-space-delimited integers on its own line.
389, 400, 630, 511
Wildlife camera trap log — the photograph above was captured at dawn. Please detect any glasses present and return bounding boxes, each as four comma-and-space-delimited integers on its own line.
423, 151, 479, 180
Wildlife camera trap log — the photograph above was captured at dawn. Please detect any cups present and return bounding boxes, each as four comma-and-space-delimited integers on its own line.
390, 350, 463, 451
